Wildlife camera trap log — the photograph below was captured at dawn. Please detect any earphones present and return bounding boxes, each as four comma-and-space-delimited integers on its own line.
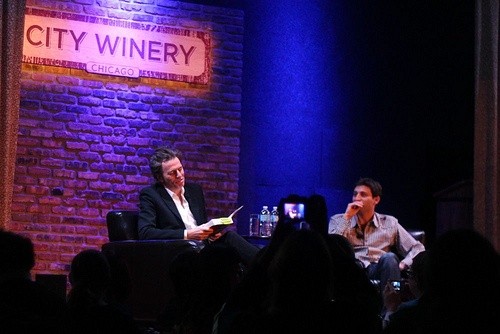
357, 234, 361, 238
383, 216, 386, 220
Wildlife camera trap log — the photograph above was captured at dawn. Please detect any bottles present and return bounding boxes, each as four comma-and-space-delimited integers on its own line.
259, 206, 272, 238
270, 207, 280, 237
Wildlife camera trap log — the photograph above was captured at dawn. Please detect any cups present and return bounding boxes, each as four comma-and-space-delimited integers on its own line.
249, 214, 260, 237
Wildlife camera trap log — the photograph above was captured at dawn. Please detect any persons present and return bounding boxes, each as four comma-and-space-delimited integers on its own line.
138, 149, 260, 276
328, 178, 426, 321
150, 229, 378, 334
381, 251, 427, 334
0, 230, 144, 334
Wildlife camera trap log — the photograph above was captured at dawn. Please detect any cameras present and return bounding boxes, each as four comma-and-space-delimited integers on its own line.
387, 279, 408, 292
279, 198, 309, 222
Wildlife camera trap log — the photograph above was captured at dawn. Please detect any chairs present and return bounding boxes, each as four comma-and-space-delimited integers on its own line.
106, 210, 138, 242
391, 230, 426, 279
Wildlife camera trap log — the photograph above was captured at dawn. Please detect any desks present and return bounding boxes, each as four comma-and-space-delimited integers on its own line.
101, 234, 270, 325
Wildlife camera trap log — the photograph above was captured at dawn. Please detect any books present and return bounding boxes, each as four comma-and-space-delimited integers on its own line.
206, 205, 244, 236
